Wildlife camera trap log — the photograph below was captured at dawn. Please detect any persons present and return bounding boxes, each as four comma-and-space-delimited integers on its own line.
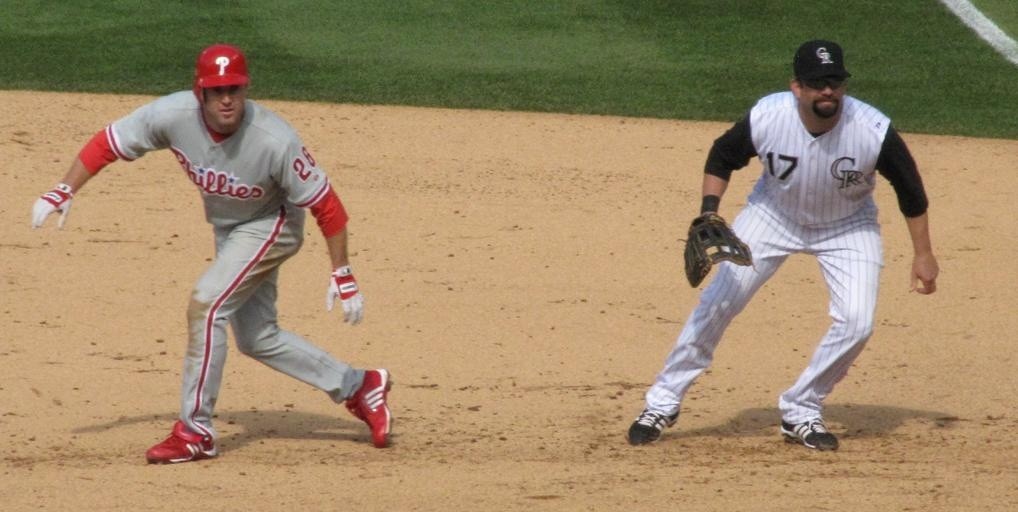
625, 39, 941, 453
28, 43, 396, 466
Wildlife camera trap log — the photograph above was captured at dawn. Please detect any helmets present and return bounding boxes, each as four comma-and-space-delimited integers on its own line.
193, 43, 249, 102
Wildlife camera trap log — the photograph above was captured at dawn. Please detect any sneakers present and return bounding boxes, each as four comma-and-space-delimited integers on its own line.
629, 409, 678, 445
145, 422, 217, 463
781, 419, 838, 449
346, 368, 394, 446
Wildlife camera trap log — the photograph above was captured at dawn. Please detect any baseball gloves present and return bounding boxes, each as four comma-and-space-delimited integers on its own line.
683, 212, 752, 288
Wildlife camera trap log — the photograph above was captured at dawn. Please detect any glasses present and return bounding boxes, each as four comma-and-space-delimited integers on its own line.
801, 79, 845, 90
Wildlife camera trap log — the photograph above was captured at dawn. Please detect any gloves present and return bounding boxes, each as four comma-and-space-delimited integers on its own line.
325, 264, 365, 323
32, 184, 72, 232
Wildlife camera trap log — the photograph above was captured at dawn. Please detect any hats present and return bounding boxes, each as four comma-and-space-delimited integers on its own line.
794, 40, 850, 78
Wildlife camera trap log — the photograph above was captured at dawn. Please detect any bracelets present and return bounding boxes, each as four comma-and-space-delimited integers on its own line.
701, 195, 722, 216
